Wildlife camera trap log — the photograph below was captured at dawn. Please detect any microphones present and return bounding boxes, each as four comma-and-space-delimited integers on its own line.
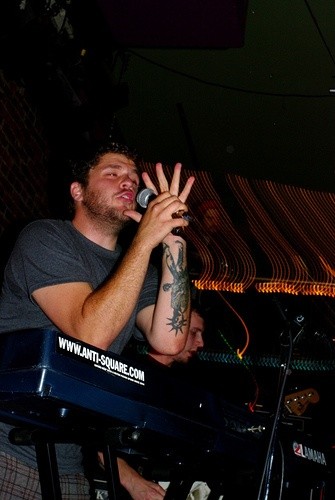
135, 188, 182, 236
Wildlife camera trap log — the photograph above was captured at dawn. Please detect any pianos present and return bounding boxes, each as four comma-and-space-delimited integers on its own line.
0, 328, 335, 488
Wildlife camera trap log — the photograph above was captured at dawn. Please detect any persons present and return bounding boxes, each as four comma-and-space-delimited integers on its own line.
97, 304, 206, 500
0, 144, 196, 500
